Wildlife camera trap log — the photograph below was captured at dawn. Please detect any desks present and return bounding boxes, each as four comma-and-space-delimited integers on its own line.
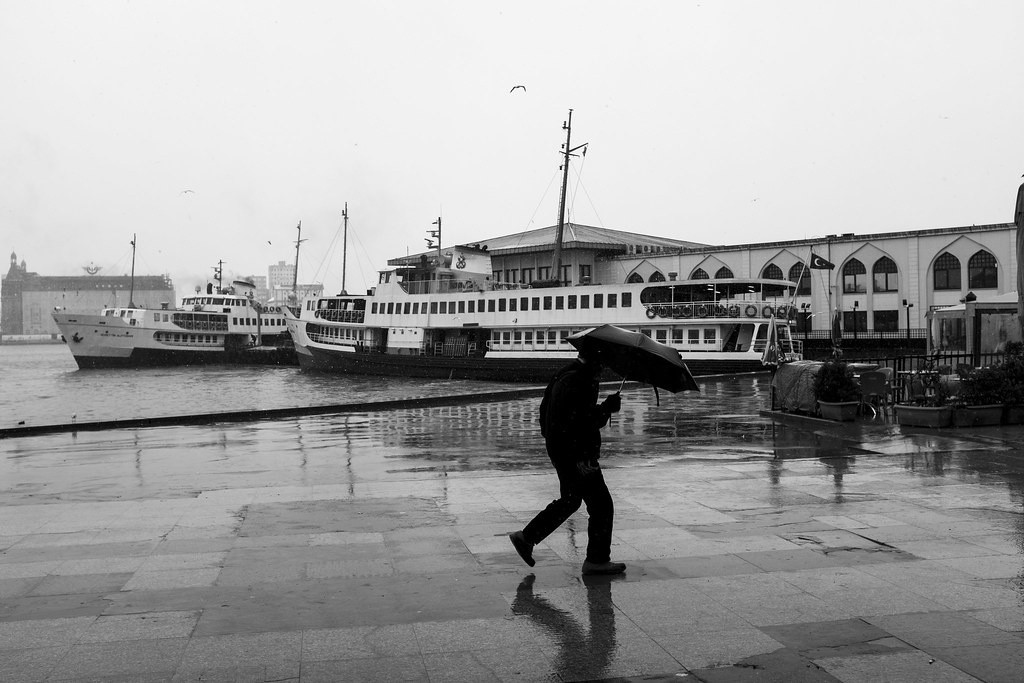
898, 370, 938, 402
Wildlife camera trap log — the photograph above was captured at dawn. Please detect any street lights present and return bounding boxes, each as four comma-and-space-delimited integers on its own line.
902, 299, 915, 352
852, 299, 861, 347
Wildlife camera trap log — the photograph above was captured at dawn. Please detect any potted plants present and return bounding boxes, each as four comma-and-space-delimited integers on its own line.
953, 369, 1004, 427
997, 340, 1024, 424
892, 382, 954, 428
817, 356, 862, 421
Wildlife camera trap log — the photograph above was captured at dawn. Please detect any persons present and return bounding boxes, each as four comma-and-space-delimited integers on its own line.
508, 338, 627, 574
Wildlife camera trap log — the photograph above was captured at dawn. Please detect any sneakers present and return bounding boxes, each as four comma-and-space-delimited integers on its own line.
581, 561, 627, 575
510, 531, 535, 567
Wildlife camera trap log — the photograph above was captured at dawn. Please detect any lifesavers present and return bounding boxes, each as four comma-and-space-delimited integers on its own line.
682, 306, 692, 317
778, 307, 787, 317
646, 308, 656, 318
713, 305, 724, 315
729, 305, 740, 317
264, 307, 268, 312
762, 306, 773, 318
180, 321, 228, 329
698, 306, 708, 317
315, 311, 364, 319
745, 305, 757, 317
672, 307, 681, 316
270, 306, 274, 311
658, 307, 668, 317
276, 307, 281, 311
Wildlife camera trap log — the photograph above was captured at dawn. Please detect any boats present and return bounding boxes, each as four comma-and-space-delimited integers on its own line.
51, 220, 309, 371
279, 106, 806, 381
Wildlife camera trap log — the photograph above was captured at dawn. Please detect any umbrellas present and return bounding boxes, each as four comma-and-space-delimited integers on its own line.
566, 322, 701, 407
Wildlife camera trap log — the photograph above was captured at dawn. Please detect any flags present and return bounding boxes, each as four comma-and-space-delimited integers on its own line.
809, 253, 835, 270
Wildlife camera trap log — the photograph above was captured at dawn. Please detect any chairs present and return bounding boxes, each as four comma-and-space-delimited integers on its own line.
935, 364, 976, 379
856, 367, 900, 423
